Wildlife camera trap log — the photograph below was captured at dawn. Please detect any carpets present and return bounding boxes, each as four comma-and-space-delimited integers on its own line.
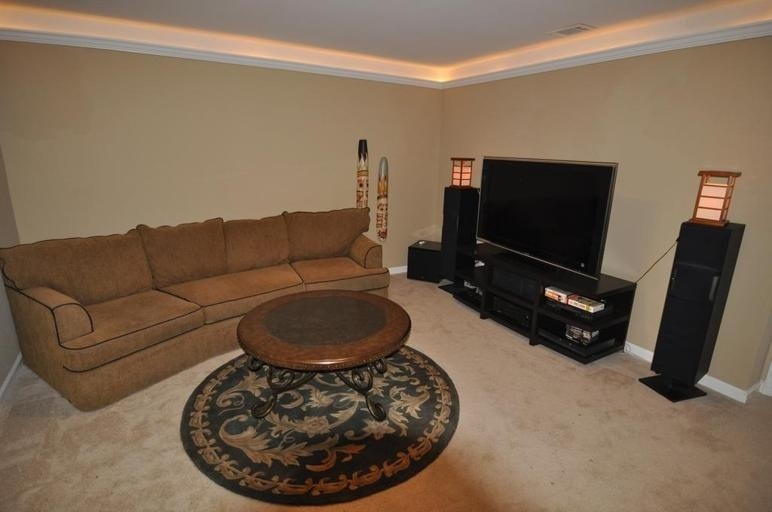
180, 345, 461, 506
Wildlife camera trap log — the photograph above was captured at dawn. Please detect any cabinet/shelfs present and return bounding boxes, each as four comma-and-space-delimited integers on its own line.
452, 243, 637, 365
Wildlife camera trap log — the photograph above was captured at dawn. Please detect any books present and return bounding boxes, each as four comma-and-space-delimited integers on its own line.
563, 323, 599, 347
544, 287, 571, 304
566, 295, 605, 313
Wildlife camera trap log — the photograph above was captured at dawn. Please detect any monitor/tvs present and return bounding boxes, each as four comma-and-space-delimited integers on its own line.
475, 155, 619, 281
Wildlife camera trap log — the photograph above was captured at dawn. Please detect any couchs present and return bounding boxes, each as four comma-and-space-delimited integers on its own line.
0, 206, 390, 413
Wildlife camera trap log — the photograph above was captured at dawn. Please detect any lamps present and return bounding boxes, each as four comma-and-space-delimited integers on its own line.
449, 157, 476, 188
689, 170, 741, 226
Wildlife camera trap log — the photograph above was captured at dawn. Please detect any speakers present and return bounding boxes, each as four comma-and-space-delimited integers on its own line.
406, 239, 441, 284
438, 184, 479, 294
637, 220, 746, 403
